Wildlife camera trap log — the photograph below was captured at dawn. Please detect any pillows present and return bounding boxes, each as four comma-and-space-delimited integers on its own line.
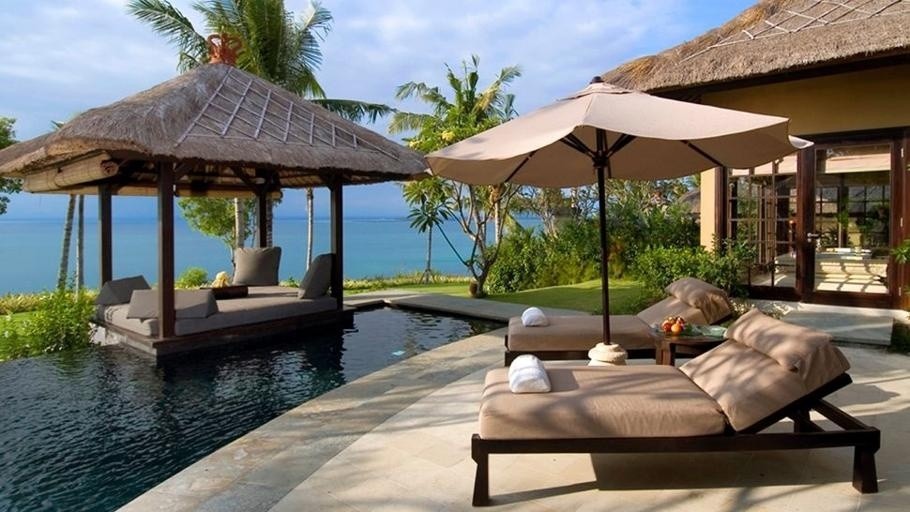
521, 305, 549, 327
200, 286, 248, 299
127, 289, 220, 320
232, 245, 281, 286
508, 354, 551, 393
95, 275, 152, 304
298, 252, 331, 299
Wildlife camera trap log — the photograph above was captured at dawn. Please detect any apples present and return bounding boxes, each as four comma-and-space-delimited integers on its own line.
661, 317, 684, 332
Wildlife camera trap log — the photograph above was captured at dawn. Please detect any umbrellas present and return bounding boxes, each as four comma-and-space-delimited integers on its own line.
426, 74, 815, 344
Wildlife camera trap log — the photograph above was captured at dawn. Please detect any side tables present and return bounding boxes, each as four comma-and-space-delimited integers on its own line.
646, 325, 728, 365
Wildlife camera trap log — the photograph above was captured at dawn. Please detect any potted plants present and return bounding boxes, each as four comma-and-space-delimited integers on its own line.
856, 216, 878, 259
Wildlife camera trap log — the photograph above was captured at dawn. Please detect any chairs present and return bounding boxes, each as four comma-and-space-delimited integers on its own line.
505, 276, 737, 366
469, 307, 880, 507
